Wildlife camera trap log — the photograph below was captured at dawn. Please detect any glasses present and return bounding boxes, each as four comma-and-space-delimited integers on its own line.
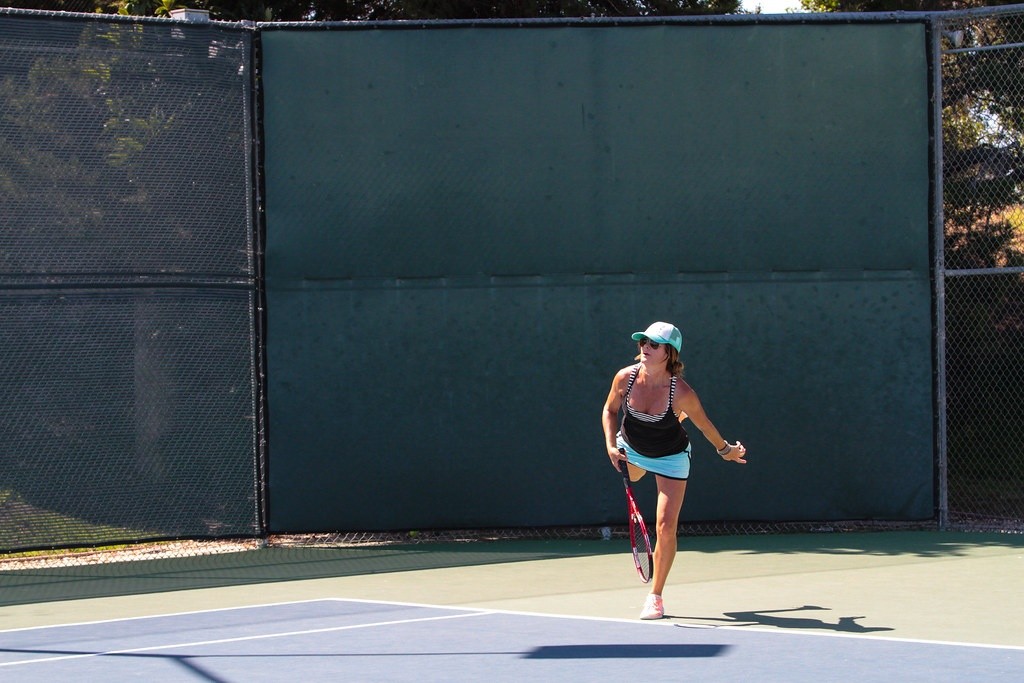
639, 338, 665, 349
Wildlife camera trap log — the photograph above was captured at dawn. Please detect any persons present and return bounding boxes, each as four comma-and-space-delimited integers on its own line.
601, 321, 747, 621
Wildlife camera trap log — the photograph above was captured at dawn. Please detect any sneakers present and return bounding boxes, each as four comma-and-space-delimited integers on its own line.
640, 595, 664, 619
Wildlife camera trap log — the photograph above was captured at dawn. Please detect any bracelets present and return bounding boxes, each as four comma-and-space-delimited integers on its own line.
716, 440, 732, 455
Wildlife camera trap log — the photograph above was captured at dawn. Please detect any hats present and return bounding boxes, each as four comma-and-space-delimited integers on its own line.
632, 322, 682, 354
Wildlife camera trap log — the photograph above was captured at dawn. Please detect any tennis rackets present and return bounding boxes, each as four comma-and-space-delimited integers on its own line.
617, 447, 654, 585
